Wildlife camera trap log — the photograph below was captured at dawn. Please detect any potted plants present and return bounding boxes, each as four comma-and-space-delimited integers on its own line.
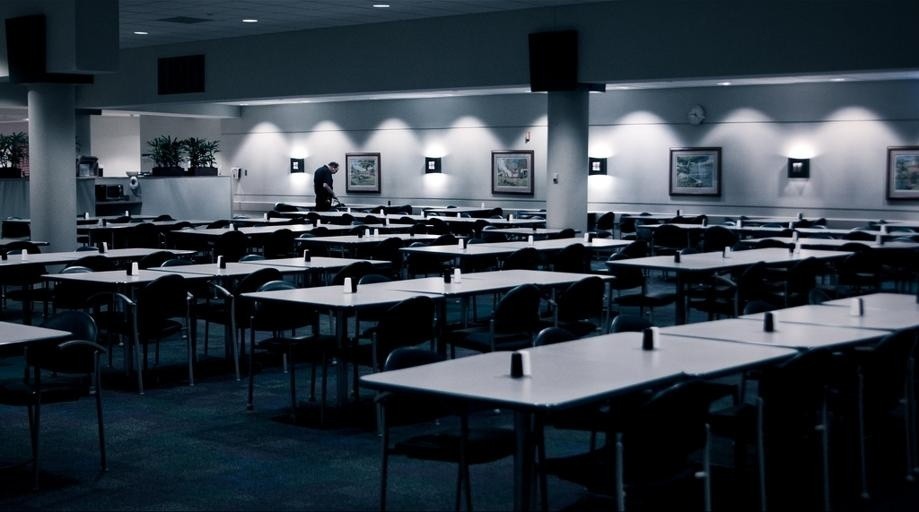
142, 133, 222, 176
0, 126, 28, 177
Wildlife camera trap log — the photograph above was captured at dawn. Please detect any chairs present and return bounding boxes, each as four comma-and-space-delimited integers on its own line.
1, 204, 919, 512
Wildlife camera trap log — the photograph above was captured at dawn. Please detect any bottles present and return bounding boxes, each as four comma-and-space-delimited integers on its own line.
508, 352, 524, 380
762, 312, 772, 333
583, 232, 592, 243
21, 248, 29, 261
1, 248, 8, 261
641, 328, 653, 352
529, 235, 534, 247
674, 208, 889, 264
303, 250, 312, 262
343, 277, 357, 293
217, 255, 226, 270
849, 295, 865, 317
443, 267, 462, 283
771, 309, 779, 331
517, 347, 531, 376
649, 326, 662, 349
83, 208, 139, 278
458, 238, 468, 250
228, 199, 514, 238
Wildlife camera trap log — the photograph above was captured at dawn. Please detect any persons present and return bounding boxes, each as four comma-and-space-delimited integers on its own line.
313, 161, 339, 211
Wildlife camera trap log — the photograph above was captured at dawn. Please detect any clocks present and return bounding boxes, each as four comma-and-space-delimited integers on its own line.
686, 103, 707, 125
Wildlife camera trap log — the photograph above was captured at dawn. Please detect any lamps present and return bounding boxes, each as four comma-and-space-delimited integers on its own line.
787, 157, 810, 178
588, 157, 607, 175
290, 157, 304, 173
425, 157, 441, 173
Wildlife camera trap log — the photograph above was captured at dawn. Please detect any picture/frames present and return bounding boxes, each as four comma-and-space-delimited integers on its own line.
490, 150, 535, 196
884, 144, 919, 202
669, 146, 722, 197
345, 152, 381, 194
